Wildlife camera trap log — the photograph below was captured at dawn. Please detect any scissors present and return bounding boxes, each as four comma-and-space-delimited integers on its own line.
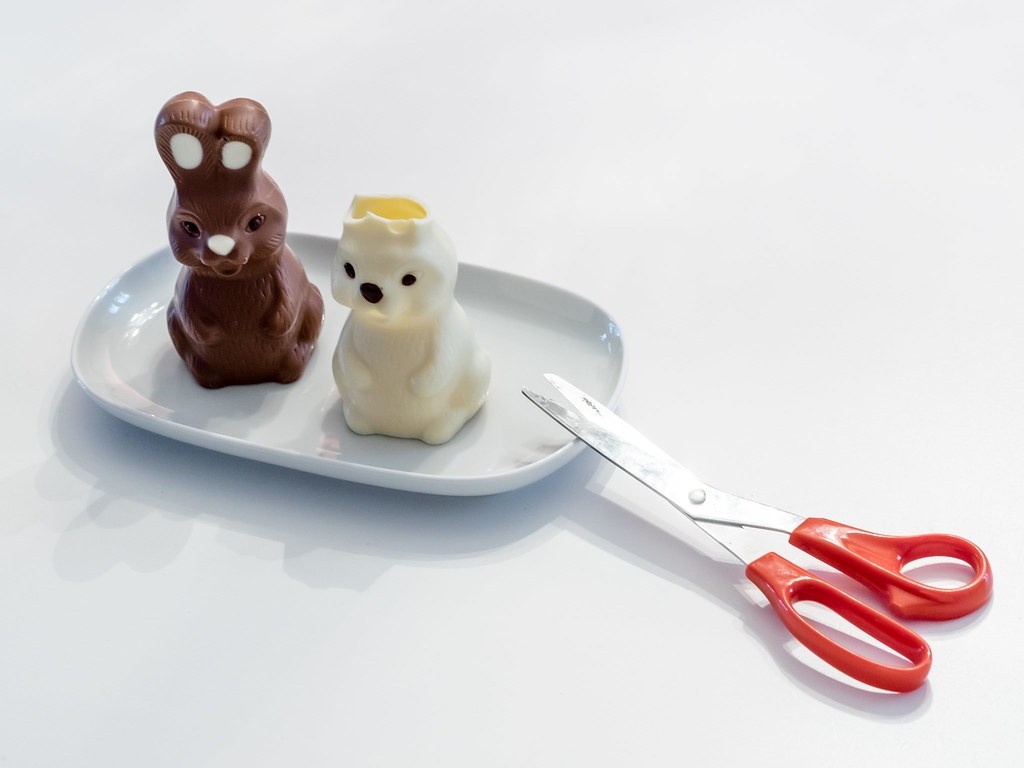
521, 368, 994, 694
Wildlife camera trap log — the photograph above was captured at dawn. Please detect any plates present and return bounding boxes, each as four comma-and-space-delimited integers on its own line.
68, 230, 626, 496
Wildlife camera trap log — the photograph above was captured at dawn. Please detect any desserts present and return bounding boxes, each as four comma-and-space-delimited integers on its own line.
156, 91, 324, 387
333, 194, 490, 443
